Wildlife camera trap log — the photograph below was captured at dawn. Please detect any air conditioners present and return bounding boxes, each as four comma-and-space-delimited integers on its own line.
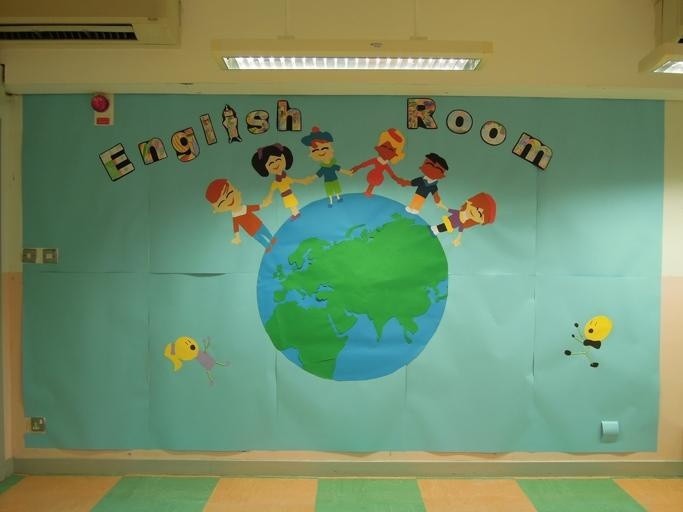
0, 1, 183, 47
